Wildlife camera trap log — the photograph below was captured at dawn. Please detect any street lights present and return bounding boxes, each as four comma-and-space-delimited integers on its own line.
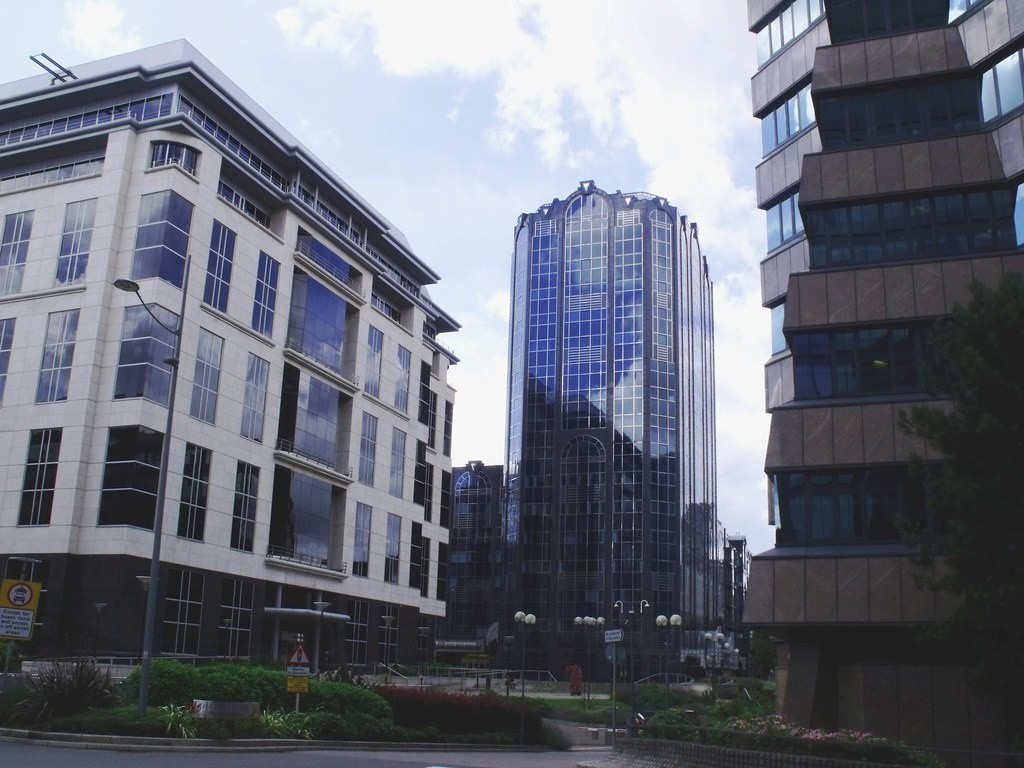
515, 611, 536, 696
93, 601, 109, 658
703, 633, 724, 693
113, 252, 194, 711
223, 618, 232, 663
613, 598, 651, 710
574, 615, 606, 704
381, 616, 396, 686
314, 602, 332, 683
417, 626, 430, 685
136, 575, 160, 662
655, 614, 682, 713
